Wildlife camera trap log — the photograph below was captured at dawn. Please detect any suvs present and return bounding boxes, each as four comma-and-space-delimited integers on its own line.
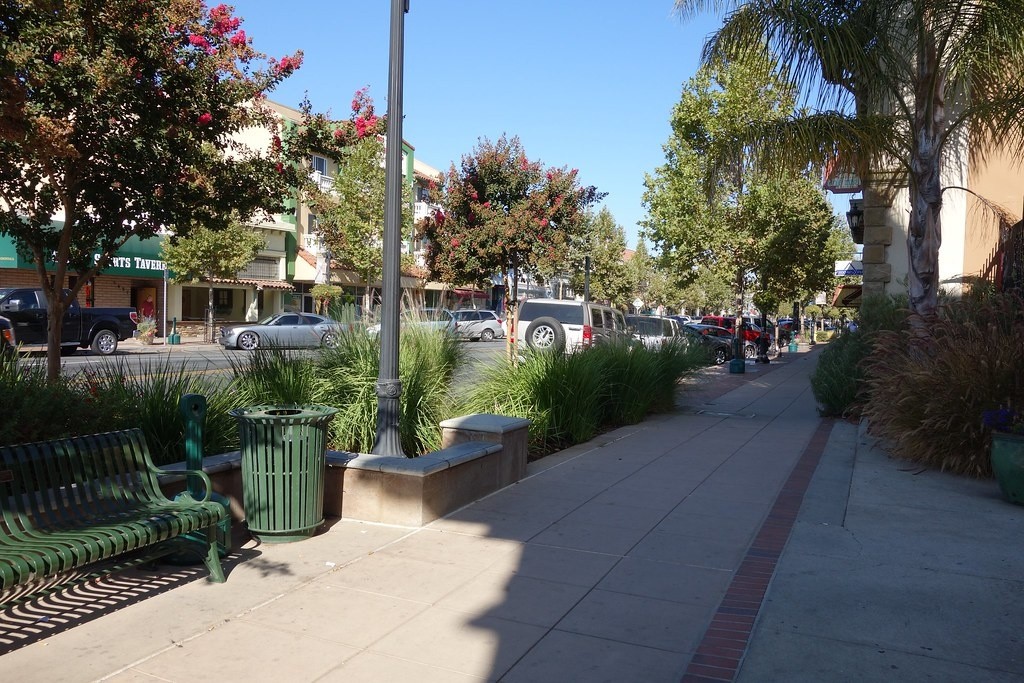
509, 298, 628, 367
624, 314, 689, 355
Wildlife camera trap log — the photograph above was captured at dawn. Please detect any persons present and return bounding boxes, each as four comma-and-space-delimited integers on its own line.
651, 303, 665, 315
843, 320, 857, 332
140, 295, 158, 339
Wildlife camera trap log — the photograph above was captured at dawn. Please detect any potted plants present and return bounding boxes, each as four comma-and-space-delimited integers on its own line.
137, 316, 157, 345
991, 418, 1024, 507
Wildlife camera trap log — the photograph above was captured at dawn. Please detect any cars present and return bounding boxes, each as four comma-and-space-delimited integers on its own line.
778, 318, 833, 336
451, 310, 504, 341
0, 316, 18, 360
218, 312, 348, 350
682, 324, 758, 365
365, 309, 459, 341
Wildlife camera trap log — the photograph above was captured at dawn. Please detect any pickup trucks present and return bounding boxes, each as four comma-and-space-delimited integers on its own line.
0, 288, 142, 357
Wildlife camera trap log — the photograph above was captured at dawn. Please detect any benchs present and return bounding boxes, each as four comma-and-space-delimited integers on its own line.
0, 427, 227, 584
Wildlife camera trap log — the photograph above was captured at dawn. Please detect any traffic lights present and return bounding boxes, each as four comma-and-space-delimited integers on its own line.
794, 302, 799, 317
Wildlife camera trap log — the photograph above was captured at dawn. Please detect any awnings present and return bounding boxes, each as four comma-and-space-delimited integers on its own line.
452, 289, 489, 299
0, 216, 192, 279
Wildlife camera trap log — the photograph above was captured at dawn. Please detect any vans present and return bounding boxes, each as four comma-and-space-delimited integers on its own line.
734, 315, 790, 347
700, 316, 771, 353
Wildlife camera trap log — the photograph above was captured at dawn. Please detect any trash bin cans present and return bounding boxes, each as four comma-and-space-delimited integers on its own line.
237, 403, 335, 544
